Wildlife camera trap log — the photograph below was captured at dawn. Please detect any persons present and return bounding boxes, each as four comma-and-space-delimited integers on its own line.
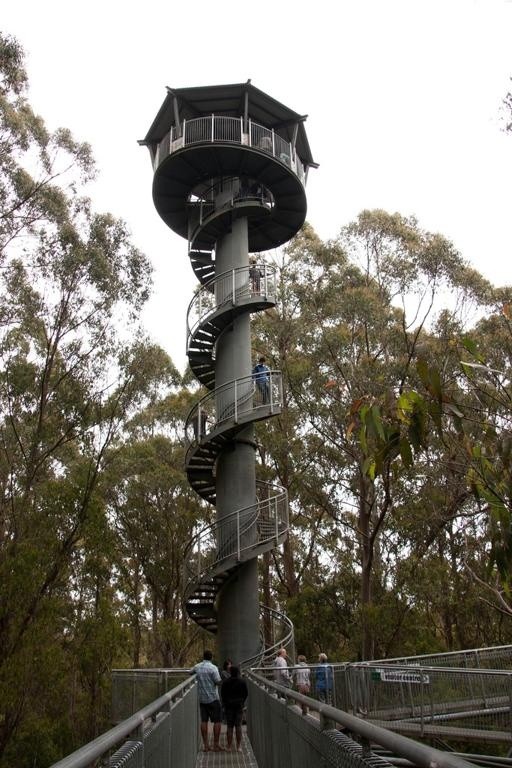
220, 657, 232, 680
250, 260, 263, 298
258, 130, 273, 152
221, 665, 247, 753
252, 357, 272, 403
279, 150, 289, 161
188, 649, 226, 753
288, 655, 313, 714
315, 652, 334, 704
272, 648, 294, 700
